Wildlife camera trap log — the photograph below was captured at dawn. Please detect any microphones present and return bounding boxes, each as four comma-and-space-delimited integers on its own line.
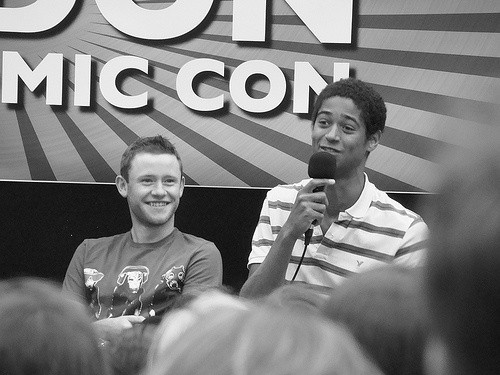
303, 151, 337, 245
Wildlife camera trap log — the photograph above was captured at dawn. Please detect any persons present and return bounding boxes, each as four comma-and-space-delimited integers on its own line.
63, 135, 222, 341
235, 76, 430, 305
0, 160, 500, 375
